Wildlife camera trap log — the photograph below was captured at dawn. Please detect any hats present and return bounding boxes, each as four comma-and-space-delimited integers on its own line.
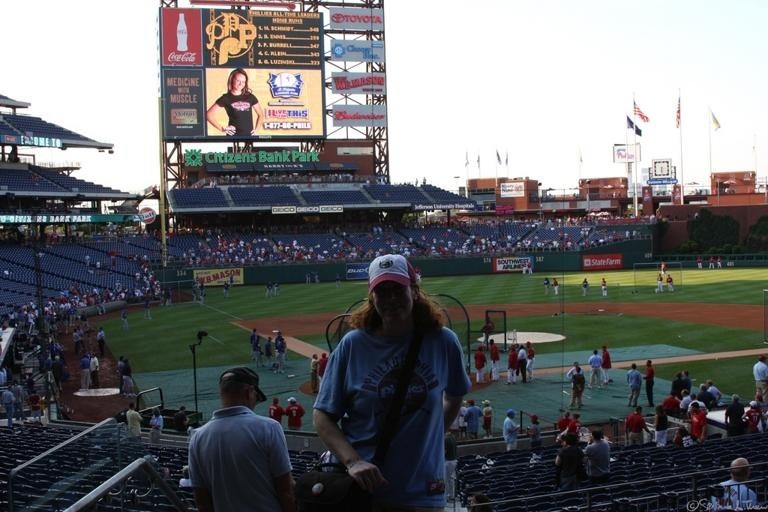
367, 253, 418, 296
219, 365, 267, 404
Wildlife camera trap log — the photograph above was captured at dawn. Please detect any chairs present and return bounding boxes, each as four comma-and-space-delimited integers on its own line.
157, 184, 615, 262
0, 418, 766, 511
0, 95, 157, 319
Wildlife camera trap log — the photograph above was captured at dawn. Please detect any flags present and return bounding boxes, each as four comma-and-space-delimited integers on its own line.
675, 96, 680, 128
464, 150, 469, 166
505, 153, 508, 166
496, 149, 501, 165
626, 114, 643, 137
634, 99, 649, 122
476, 155, 480, 167
710, 109, 722, 133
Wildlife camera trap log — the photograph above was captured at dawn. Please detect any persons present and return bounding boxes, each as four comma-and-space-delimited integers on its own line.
206, 68, 265, 136
601, 278, 607, 292
551, 278, 559, 296
716, 256, 721, 269
656, 273, 663, 293
311, 252, 473, 510
602, 281, 607, 296
527, 262, 532, 275
521, 262, 526, 274
181, 324, 767, 475
186, 365, 296, 512
666, 274, 674, 292
708, 257, 714, 269
1, 213, 703, 269
543, 278, 550, 295
661, 261, 665, 274
581, 278, 589, 296
710, 457, 758, 510
696, 256, 703, 269
